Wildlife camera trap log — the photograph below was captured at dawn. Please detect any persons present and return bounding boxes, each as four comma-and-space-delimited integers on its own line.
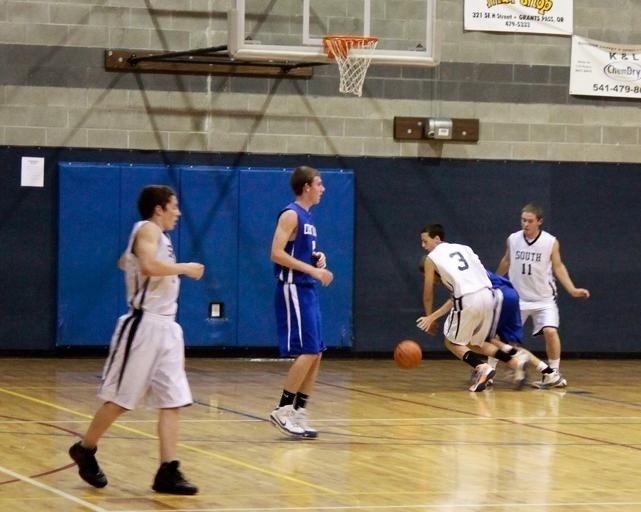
495, 201, 591, 391
417, 249, 562, 391
415, 222, 529, 395
67, 182, 205, 495
270, 163, 334, 441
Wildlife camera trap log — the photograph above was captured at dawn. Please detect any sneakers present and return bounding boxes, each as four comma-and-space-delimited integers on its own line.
468, 359, 568, 392
153, 460, 197, 495
69, 441, 107, 488
269, 404, 317, 438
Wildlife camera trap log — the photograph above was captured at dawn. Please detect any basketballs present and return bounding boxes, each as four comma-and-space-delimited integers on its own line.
394, 340, 422, 370
